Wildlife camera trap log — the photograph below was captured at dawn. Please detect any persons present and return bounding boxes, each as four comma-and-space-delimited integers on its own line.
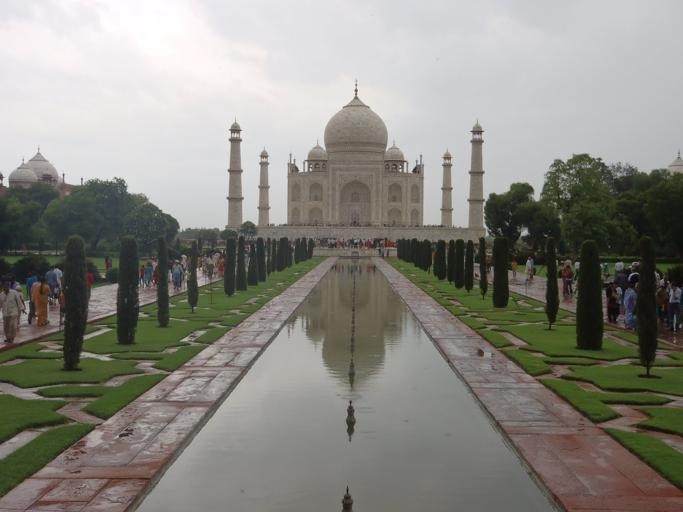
486, 255, 491, 273
510, 255, 683, 331
314, 236, 399, 248
0, 251, 227, 343
